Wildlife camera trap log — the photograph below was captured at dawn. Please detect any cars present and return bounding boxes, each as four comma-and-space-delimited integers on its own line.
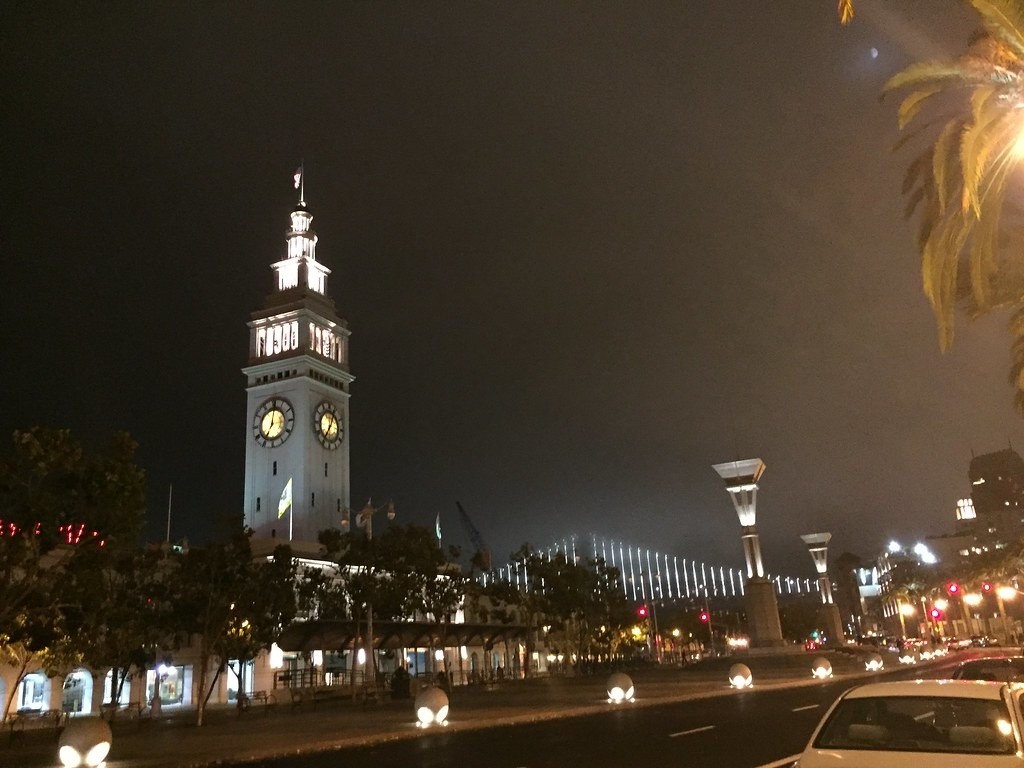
790, 656, 1024, 768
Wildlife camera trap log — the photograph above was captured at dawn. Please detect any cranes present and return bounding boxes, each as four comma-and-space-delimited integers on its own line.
454, 500, 493, 574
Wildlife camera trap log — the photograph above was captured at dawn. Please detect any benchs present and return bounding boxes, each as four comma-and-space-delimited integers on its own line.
236, 691, 278, 719
100, 703, 152, 736
7, 709, 70, 746
835, 720, 1010, 754
289, 687, 335, 713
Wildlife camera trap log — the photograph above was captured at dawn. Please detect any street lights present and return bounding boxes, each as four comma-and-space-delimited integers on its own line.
342, 496, 395, 542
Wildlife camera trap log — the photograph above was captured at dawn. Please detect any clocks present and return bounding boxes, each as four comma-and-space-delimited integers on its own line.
253, 398, 295, 448
314, 402, 344, 451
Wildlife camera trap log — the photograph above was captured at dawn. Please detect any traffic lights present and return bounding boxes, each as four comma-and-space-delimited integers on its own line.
949, 583, 959, 594
812, 632, 819, 638
931, 610, 939, 617
699, 612, 710, 622
637, 608, 648, 617
982, 583, 993, 593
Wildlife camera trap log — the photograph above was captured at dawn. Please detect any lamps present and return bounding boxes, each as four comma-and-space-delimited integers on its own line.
920, 643, 949, 660
864, 653, 884, 672
606, 672, 635, 704
812, 656, 833, 680
729, 663, 754, 690
414, 687, 449, 728
57, 716, 112, 768
899, 644, 917, 665
